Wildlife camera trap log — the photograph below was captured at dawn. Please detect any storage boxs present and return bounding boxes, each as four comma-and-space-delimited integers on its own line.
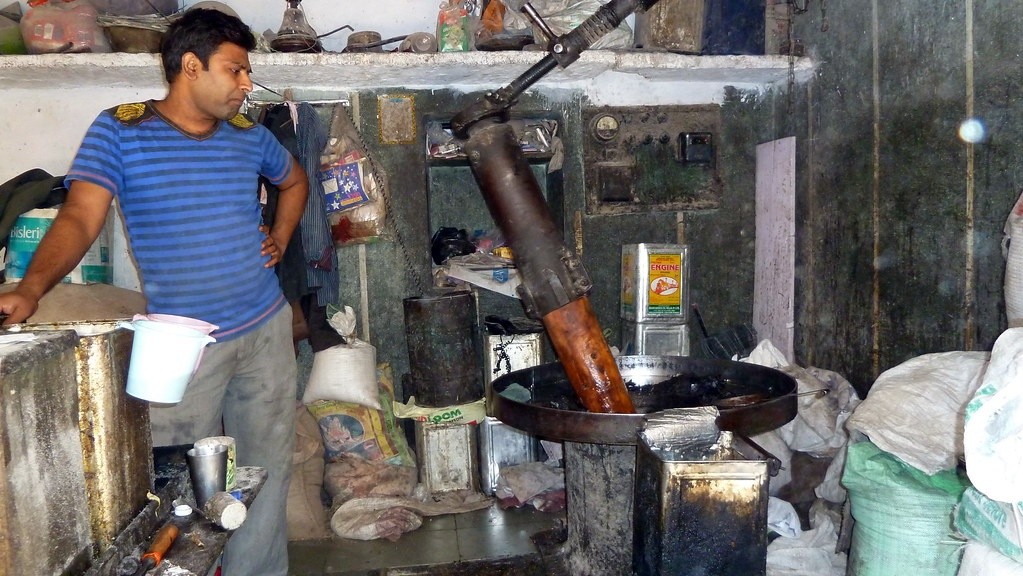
616, 240, 692, 328
618, 316, 693, 360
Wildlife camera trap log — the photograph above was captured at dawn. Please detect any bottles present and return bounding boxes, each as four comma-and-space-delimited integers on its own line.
398, 32, 437, 54
437, 0, 469, 53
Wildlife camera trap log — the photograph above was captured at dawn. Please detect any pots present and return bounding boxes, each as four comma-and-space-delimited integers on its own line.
489, 355, 830, 446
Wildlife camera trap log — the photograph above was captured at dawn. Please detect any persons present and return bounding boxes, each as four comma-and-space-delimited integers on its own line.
0, 8, 309, 576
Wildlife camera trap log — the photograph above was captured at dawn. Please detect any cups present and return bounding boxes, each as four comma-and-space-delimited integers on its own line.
204, 491, 247, 530
194, 436, 237, 491
126, 320, 216, 403
186, 445, 228, 507
133, 314, 219, 386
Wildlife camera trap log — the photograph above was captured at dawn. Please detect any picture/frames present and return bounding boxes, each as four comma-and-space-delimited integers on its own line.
373, 92, 419, 148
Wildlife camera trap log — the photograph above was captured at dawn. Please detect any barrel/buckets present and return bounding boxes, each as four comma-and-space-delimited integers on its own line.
403, 290, 486, 408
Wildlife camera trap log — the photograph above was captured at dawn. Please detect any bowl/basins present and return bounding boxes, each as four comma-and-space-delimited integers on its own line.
100, 24, 165, 54
